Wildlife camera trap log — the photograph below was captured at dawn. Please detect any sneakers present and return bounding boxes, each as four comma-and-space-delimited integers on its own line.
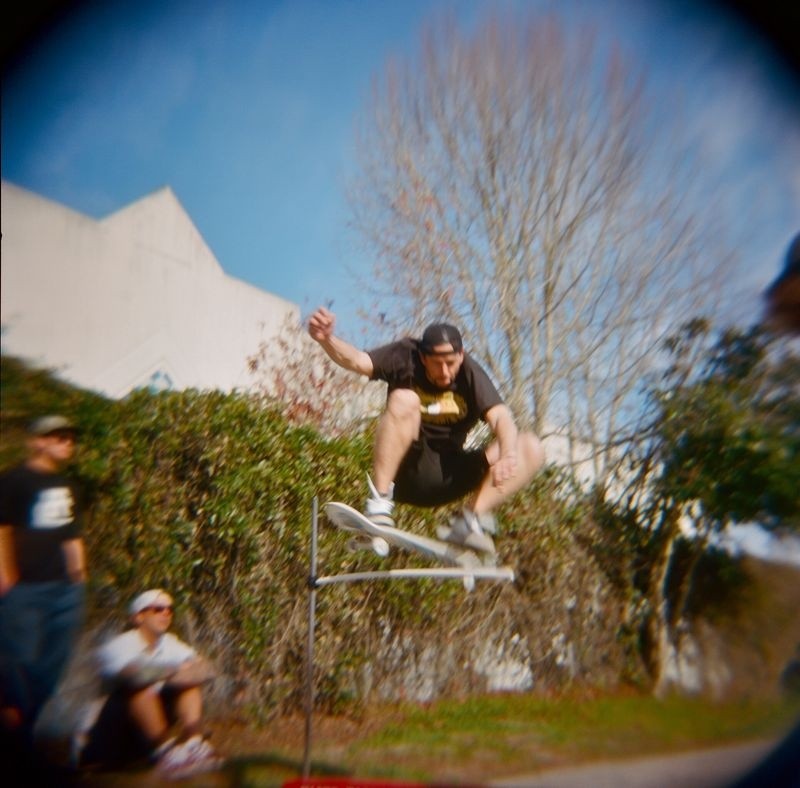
435, 504, 497, 553
159, 736, 227, 777
363, 472, 398, 527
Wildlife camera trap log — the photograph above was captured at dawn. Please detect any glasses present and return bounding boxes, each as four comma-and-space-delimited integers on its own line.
45, 434, 81, 445
141, 604, 178, 613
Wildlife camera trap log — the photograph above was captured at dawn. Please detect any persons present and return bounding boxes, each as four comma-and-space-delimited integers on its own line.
1, 413, 93, 787
306, 306, 546, 555
95, 587, 223, 776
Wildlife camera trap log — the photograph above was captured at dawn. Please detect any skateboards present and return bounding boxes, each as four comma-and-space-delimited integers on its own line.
322, 500, 496, 590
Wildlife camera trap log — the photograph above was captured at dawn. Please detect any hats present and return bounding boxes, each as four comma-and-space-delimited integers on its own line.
28, 417, 85, 436
418, 323, 462, 356
127, 589, 175, 612
